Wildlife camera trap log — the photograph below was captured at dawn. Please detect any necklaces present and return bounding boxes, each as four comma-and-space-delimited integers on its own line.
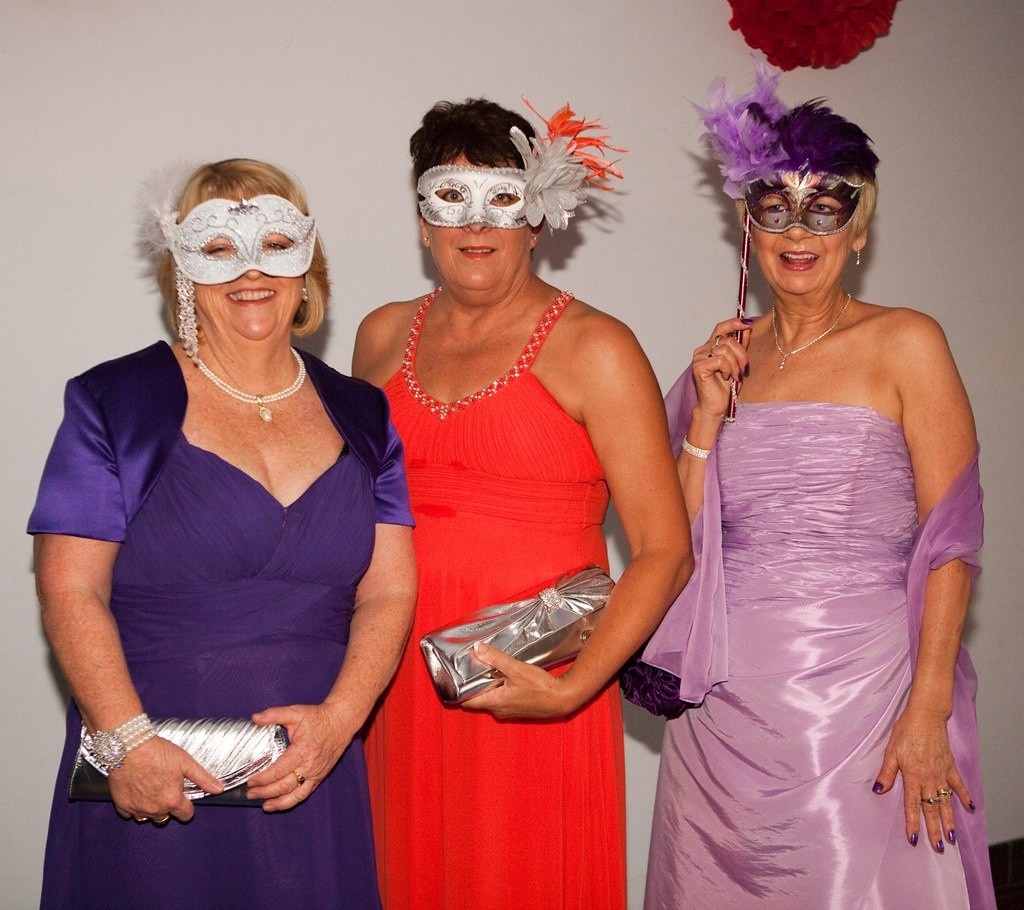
197, 346, 307, 424
770, 292, 853, 372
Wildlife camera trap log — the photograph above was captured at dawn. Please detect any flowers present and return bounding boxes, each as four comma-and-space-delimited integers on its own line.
727, 0, 898, 73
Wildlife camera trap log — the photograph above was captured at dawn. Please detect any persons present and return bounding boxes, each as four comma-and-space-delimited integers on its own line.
346, 95, 699, 910
619, 53, 1003, 910
23, 154, 420, 910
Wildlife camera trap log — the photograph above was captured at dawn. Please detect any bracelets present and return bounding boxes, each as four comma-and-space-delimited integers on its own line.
85, 711, 159, 770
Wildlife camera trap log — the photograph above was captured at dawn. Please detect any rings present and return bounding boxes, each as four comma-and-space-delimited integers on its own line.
134, 815, 146, 823
154, 815, 170, 825
291, 768, 305, 786
921, 796, 942, 804
937, 785, 953, 796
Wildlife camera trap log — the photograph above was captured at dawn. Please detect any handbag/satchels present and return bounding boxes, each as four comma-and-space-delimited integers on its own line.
404, 560, 636, 709
61, 707, 296, 817
